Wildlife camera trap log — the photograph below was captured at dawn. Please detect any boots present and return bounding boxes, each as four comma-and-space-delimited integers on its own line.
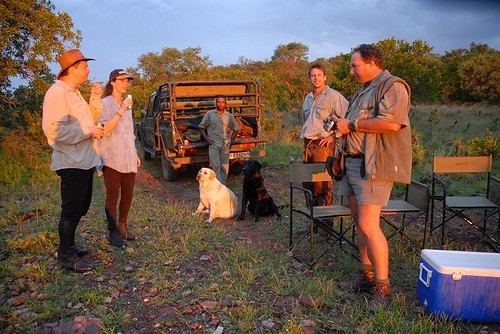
364, 280, 390, 312
75, 245, 89, 256
59, 218, 94, 273
338, 264, 374, 291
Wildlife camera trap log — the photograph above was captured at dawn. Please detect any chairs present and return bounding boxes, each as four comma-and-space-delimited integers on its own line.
429, 154, 500, 252
351, 179, 430, 251
288, 160, 362, 270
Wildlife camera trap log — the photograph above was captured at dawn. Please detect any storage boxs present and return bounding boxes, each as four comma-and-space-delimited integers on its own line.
415, 249, 500, 324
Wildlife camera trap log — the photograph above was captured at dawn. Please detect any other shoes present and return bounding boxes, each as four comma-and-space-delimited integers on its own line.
111, 231, 127, 247
118, 223, 135, 240
313, 227, 328, 242
297, 226, 310, 234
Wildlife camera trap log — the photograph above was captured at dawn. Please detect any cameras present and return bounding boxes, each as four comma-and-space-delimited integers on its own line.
324, 113, 340, 131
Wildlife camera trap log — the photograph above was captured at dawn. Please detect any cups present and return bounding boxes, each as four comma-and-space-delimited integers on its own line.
184, 140, 189, 146
128, 93, 133, 109
93, 123, 104, 139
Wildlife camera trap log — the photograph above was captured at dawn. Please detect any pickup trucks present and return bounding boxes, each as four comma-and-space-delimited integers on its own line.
135, 78, 266, 181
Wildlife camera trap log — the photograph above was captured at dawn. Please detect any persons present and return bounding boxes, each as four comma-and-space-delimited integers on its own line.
94, 70, 141, 248
299, 64, 349, 236
333, 44, 412, 311
198, 97, 239, 187
42, 50, 105, 271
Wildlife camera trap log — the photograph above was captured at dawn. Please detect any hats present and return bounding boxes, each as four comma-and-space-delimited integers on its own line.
110, 69, 134, 81
58, 49, 95, 76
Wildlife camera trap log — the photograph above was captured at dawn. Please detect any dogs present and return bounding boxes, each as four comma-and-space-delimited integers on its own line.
191, 167, 239, 223
237, 159, 281, 221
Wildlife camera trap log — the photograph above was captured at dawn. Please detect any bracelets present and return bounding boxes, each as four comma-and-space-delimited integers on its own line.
116, 111, 122, 118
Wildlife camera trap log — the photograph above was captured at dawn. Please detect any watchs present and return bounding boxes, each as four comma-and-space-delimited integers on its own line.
349, 119, 358, 133
